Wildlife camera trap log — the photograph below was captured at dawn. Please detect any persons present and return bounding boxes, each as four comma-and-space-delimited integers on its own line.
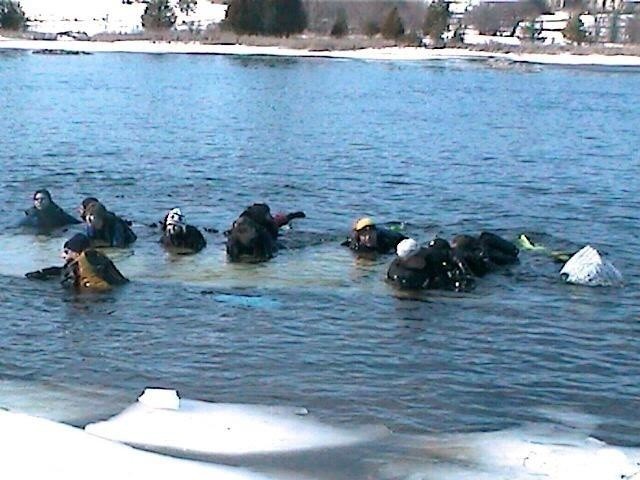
337, 215, 519, 293
25, 233, 130, 290
25, 188, 82, 228
160, 207, 206, 256
86, 201, 138, 248
224, 202, 306, 263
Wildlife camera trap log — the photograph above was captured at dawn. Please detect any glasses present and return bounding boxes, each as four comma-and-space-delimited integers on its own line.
64, 250, 69, 256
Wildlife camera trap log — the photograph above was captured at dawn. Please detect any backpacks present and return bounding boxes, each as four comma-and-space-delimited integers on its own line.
68, 250, 129, 288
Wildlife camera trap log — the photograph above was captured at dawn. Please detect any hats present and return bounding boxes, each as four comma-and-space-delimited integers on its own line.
396, 236, 421, 263
354, 218, 377, 232
64, 232, 89, 252
165, 206, 186, 232
